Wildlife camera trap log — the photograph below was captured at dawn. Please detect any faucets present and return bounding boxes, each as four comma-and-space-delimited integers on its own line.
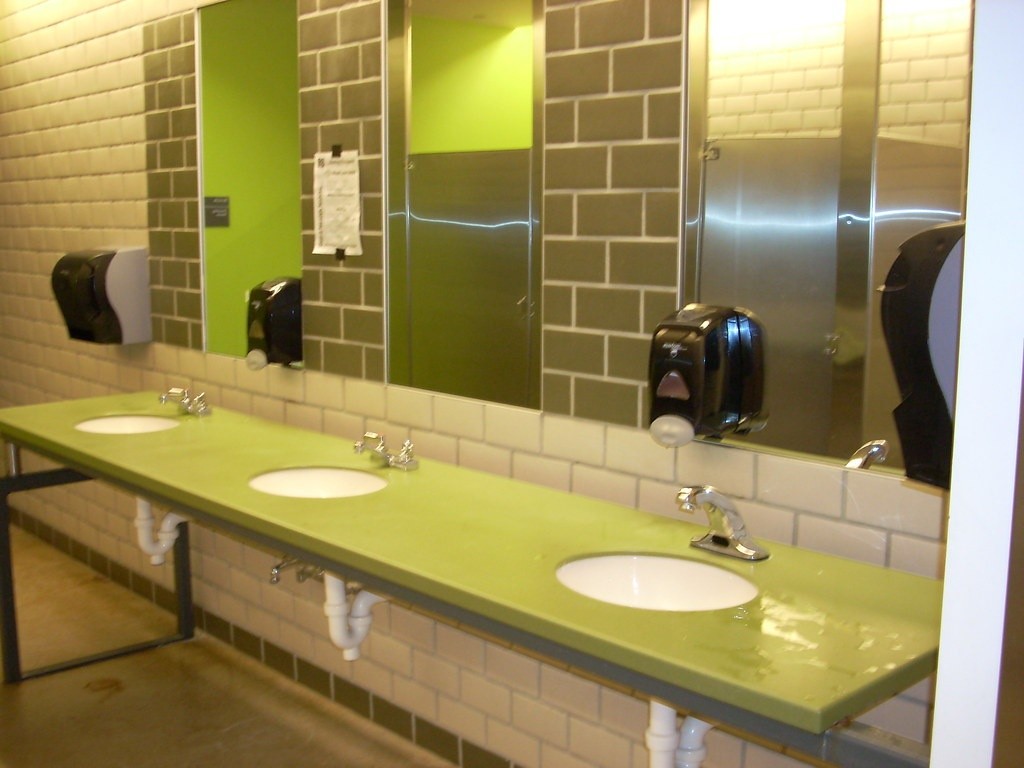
354, 440, 389, 468
677, 481, 773, 562
844, 435, 891, 471
157, 390, 194, 417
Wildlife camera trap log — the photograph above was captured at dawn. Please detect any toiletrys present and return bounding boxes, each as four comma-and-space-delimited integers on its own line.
244, 277, 303, 372
644, 299, 741, 448
737, 308, 772, 435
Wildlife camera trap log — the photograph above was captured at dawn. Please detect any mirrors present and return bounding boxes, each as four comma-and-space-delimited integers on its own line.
379, 0, 548, 415
674, 0, 977, 479
194, 0, 303, 358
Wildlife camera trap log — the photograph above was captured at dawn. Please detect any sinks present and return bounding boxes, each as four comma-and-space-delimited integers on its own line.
73, 410, 180, 435
245, 461, 391, 499
551, 546, 763, 614
